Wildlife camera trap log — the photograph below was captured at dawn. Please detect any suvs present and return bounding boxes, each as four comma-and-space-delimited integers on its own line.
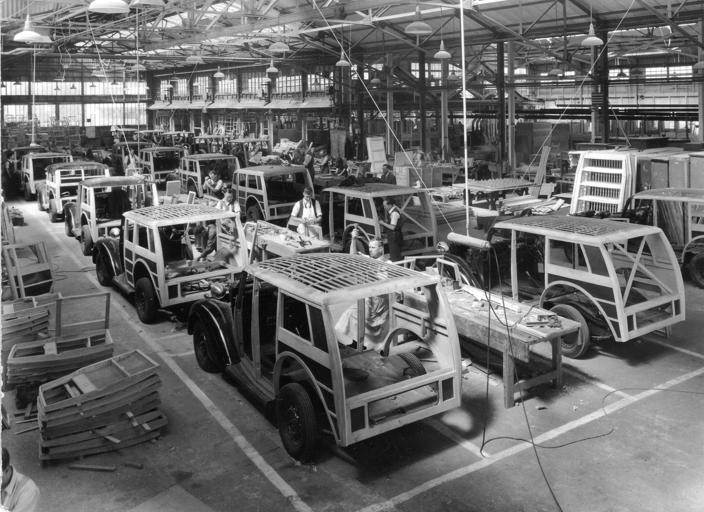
62, 175, 147, 255
287, 183, 439, 270
105, 127, 275, 198
36, 159, 111, 224
223, 163, 315, 227
565, 187, 703, 288
9, 145, 73, 201
436, 214, 686, 360
94, 200, 248, 324
185, 250, 470, 460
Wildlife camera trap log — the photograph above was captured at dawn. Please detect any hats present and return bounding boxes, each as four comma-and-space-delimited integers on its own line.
303, 187, 312, 197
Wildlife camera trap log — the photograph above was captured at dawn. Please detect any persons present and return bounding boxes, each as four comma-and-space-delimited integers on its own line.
1, 446, 40, 512
49, 107, 638, 287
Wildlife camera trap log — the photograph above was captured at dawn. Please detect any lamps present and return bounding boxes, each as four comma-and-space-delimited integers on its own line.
88, 1, 130, 13
579, 1, 604, 50
434, 4, 452, 59
403, 3, 433, 38
13, 0, 53, 45
264, 56, 279, 77
268, 24, 290, 55
211, 64, 225, 80
335, 27, 350, 66
128, 0, 167, 9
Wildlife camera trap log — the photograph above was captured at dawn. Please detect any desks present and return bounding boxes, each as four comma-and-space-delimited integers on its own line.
159, 193, 202, 204
243, 220, 331, 258
392, 270, 581, 408
454, 178, 535, 210
